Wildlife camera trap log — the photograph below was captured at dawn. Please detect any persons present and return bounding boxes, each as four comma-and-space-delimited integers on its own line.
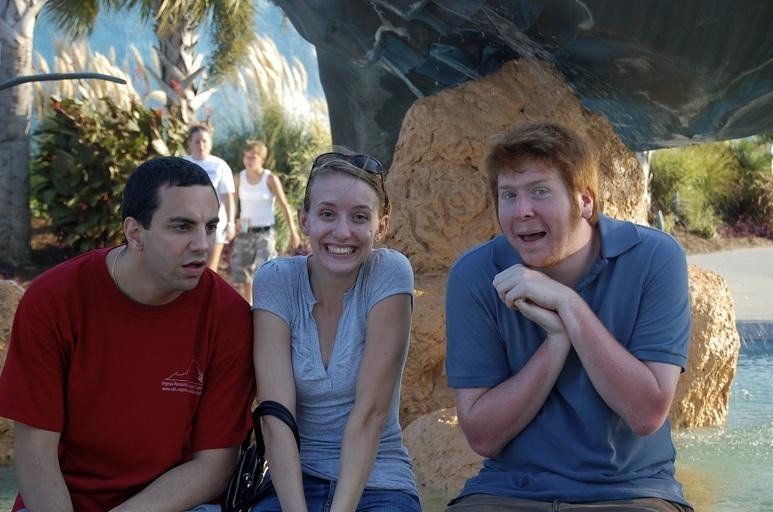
177, 125, 236, 276
0, 154, 265, 512
243, 144, 423, 511
228, 138, 301, 306
441, 121, 697, 512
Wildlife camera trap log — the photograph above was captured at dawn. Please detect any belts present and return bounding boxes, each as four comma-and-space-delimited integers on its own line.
250, 224, 275, 233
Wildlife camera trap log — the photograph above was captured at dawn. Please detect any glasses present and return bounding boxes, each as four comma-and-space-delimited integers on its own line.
306, 153, 390, 209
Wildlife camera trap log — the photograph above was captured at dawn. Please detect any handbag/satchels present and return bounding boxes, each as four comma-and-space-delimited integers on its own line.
222, 400, 301, 512
